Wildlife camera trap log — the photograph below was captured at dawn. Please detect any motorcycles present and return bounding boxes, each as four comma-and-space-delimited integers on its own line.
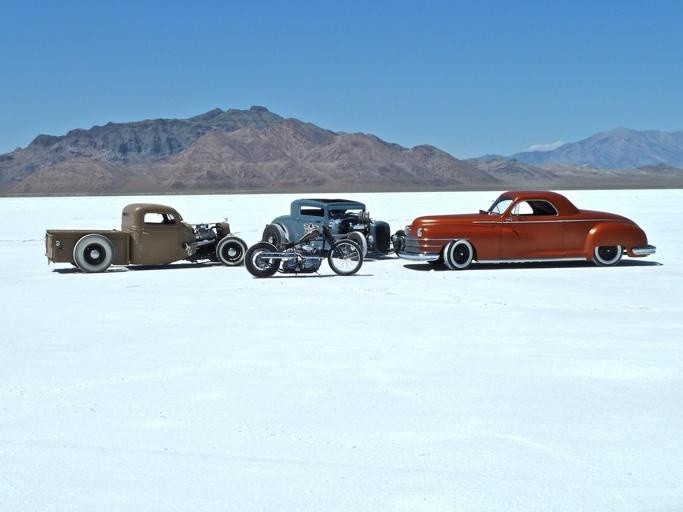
245, 224, 363, 277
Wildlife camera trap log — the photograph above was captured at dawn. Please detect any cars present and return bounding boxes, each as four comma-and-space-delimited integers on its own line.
262, 199, 390, 255
390, 190, 656, 270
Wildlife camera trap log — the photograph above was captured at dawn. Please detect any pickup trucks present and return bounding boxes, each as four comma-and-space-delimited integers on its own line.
46, 203, 248, 272
